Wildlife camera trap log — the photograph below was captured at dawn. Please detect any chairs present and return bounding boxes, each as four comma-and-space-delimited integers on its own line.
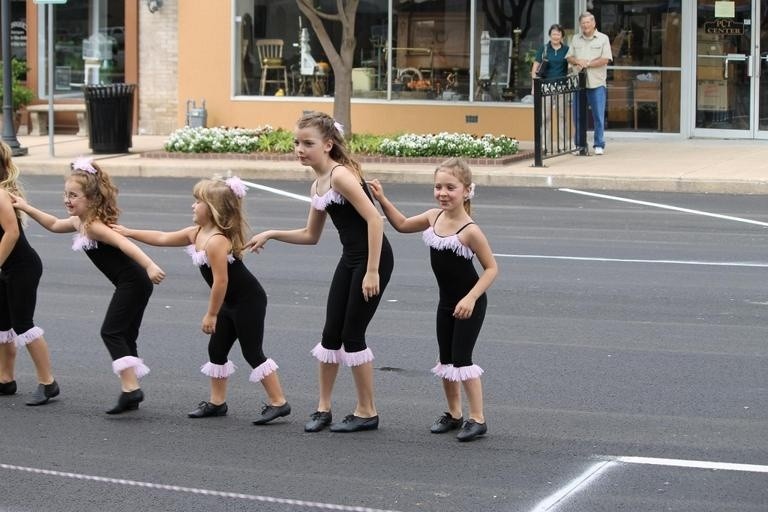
256, 39, 289, 96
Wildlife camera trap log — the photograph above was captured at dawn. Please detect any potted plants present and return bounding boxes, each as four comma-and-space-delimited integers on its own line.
0, 57, 36, 134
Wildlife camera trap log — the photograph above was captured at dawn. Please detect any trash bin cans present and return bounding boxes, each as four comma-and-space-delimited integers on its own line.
83, 83, 136, 155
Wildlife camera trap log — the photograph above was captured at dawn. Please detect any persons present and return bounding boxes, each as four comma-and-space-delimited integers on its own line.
0, 138, 61, 406
106, 176, 291, 425
364, 156, 498, 442
564, 11, 613, 156
7, 159, 166, 415
531, 24, 569, 97
241, 111, 393, 434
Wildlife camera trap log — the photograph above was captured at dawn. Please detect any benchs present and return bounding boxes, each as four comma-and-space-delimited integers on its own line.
27, 104, 88, 135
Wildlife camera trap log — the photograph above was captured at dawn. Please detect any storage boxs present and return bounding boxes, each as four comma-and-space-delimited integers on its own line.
608, 32, 736, 131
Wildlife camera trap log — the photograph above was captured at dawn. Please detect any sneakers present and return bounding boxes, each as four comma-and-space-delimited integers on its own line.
570, 147, 588, 156
594, 146, 605, 156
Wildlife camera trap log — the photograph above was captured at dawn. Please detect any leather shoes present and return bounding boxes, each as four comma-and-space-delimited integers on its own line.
456, 418, 487, 441
23, 375, 60, 406
105, 389, 144, 416
188, 397, 229, 419
328, 412, 379, 433
1, 379, 18, 398
429, 412, 465, 434
252, 399, 292, 426
304, 410, 333, 433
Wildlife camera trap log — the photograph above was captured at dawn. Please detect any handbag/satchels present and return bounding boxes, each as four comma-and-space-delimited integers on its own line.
533, 45, 551, 79
